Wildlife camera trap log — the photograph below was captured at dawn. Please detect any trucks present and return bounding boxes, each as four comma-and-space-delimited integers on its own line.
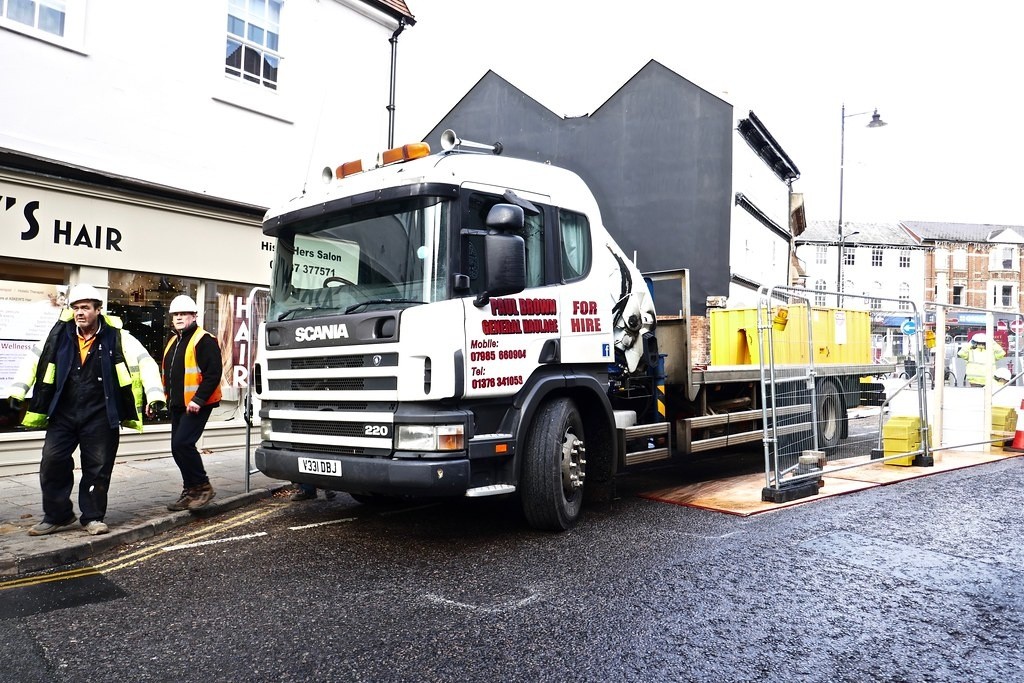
252, 128, 898, 537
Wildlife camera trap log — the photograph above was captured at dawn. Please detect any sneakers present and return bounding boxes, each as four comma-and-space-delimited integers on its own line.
167, 484, 202, 511
187, 481, 217, 511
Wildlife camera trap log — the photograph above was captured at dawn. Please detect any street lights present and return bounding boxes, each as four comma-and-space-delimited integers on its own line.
837, 109, 888, 308
842, 232, 861, 307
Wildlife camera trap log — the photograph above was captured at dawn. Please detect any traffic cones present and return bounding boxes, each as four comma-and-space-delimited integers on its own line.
1002, 398, 1024, 453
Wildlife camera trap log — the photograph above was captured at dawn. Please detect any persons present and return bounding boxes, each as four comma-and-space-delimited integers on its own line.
159, 295, 222, 512
9, 283, 166, 536
957, 332, 1012, 388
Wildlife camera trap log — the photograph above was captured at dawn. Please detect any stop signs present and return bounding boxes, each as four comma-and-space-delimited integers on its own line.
1010, 319, 1023, 334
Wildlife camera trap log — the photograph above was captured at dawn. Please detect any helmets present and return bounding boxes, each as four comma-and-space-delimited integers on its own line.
973, 333, 986, 343
68, 284, 103, 305
169, 295, 197, 313
994, 368, 1012, 381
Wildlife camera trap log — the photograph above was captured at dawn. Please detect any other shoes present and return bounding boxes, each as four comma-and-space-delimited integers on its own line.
27, 515, 79, 535
78, 520, 110, 534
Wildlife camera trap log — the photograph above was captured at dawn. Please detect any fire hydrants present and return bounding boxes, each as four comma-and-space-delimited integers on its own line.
1007, 360, 1014, 375
